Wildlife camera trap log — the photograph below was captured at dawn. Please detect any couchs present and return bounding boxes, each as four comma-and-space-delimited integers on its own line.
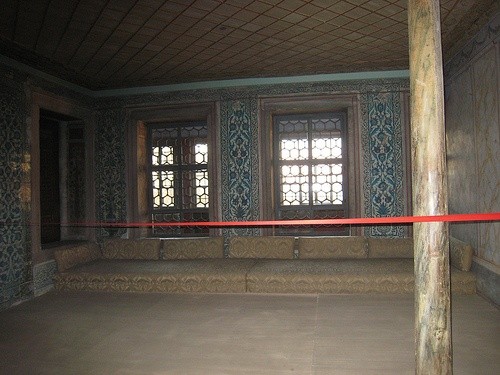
56, 235, 476, 296
